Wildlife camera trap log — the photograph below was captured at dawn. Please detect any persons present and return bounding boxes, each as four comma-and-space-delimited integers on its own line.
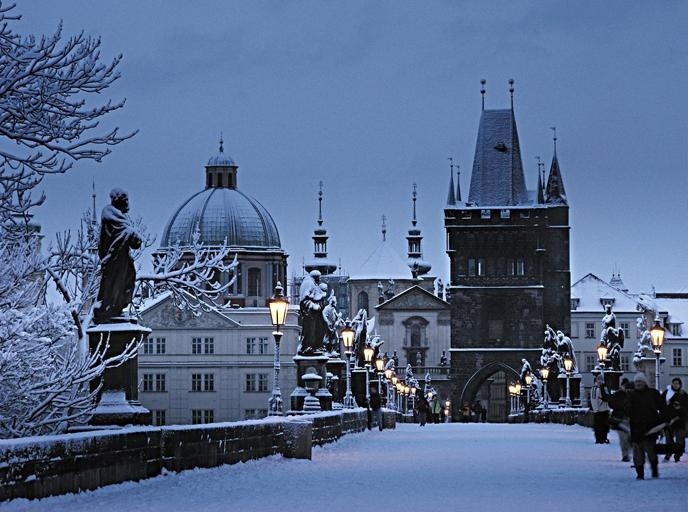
590, 374, 610, 443
520, 330, 576, 385
660, 376, 688, 462
320, 294, 340, 356
608, 378, 632, 461
96, 187, 143, 320
621, 372, 662, 479
431, 394, 442, 423
606, 329, 620, 362
600, 301, 617, 347
480, 405, 488, 423
349, 308, 432, 394
297, 267, 327, 356
475, 400, 483, 423
414, 393, 429, 426
461, 400, 472, 424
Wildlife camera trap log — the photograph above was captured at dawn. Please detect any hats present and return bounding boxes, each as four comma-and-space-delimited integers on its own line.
633, 373, 647, 383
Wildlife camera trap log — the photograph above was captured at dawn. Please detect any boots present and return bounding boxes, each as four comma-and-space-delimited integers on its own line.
635, 464, 659, 480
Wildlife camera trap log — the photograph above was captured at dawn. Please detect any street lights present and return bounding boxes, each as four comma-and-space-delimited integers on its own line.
364, 349, 374, 408
268, 281, 288, 416
564, 359, 572, 407
375, 359, 417, 413
341, 327, 356, 409
541, 369, 549, 409
651, 318, 665, 391
525, 374, 532, 411
509, 380, 521, 412
597, 345, 608, 381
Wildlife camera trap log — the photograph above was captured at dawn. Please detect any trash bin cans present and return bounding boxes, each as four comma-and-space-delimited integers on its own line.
383, 411, 396, 429
284, 420, 312, 459
